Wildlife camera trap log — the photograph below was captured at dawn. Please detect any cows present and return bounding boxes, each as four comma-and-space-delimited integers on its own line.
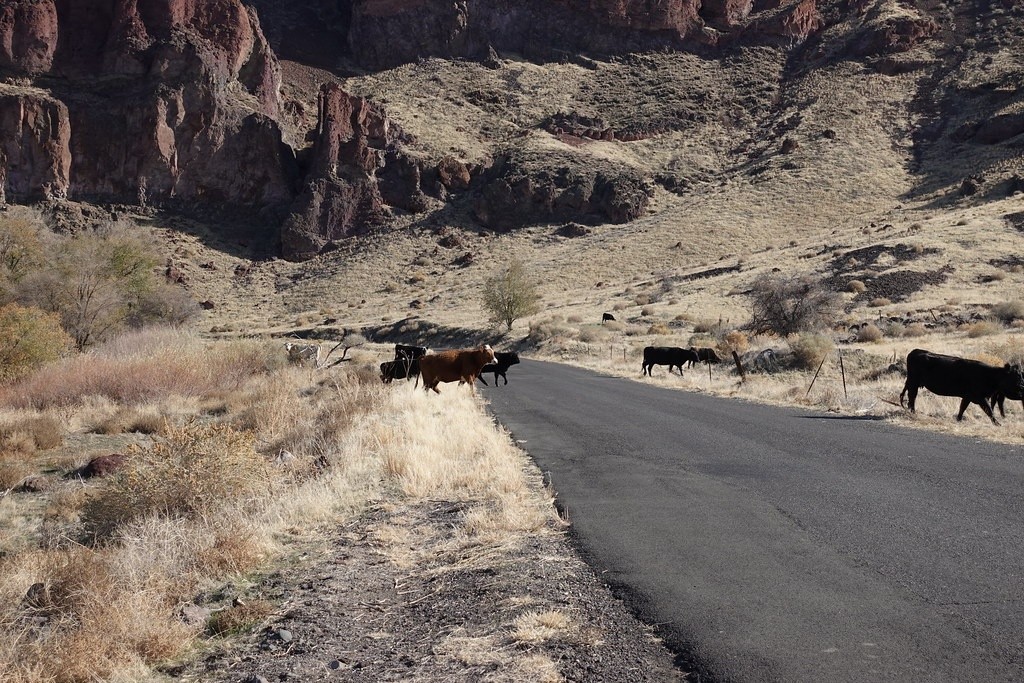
640, 346, 721, 379
602, 312, 616, 324
897, 349, 1024, 427
379, 344, 520, 395
283, 342, 322, 368
